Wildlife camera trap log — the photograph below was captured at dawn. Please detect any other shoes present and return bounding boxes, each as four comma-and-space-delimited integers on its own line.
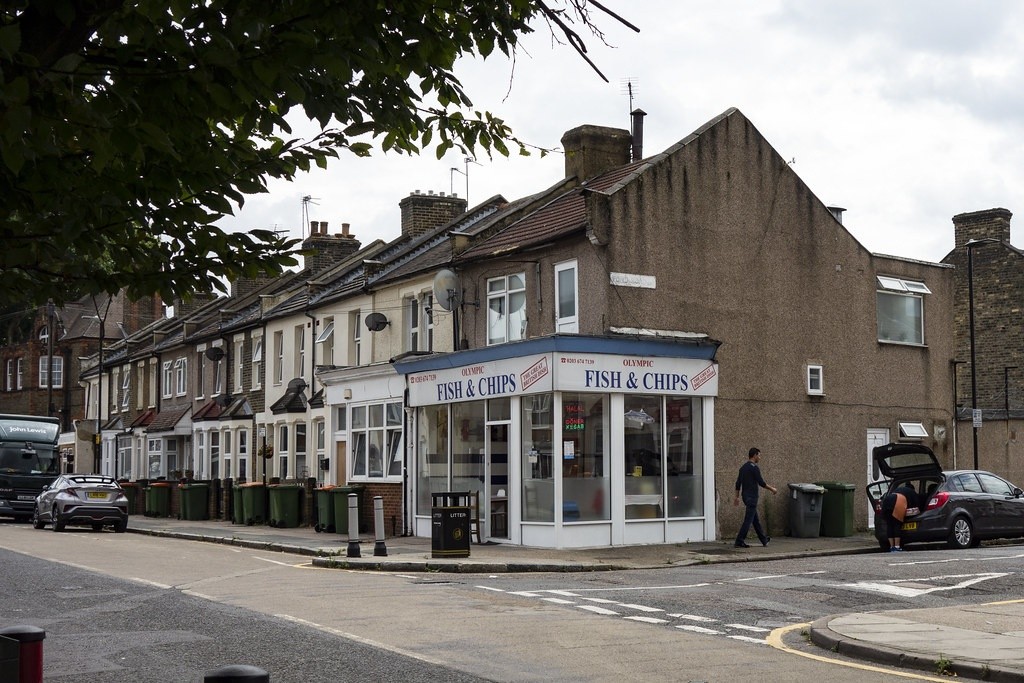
764, 536, 770, 546
890, 546, 903, 552
735, 543, 750, 548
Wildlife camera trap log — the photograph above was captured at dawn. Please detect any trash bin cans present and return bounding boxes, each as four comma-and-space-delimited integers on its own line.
430, 491, 472, 558
119, 482, 209, 520
231, 481, 268, 526
813, 481, 856, 537
267, 484, 300, 527
785, 482, 828, 538
312, 485, 365, 533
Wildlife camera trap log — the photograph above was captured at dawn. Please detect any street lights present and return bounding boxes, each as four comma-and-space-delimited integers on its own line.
81, 315, 103, 474
964, 235, 1000, 470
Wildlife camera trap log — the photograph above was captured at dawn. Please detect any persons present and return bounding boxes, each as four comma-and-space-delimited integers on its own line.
733, 448, 777, 548
880, 482, 917, 552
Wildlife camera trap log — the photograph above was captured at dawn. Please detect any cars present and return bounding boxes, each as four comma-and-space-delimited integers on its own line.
32, 473, 129, 533
864, 441, 1024, 549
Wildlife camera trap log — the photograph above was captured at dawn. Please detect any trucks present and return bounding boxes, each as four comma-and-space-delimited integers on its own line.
0, 414, 62, 522
